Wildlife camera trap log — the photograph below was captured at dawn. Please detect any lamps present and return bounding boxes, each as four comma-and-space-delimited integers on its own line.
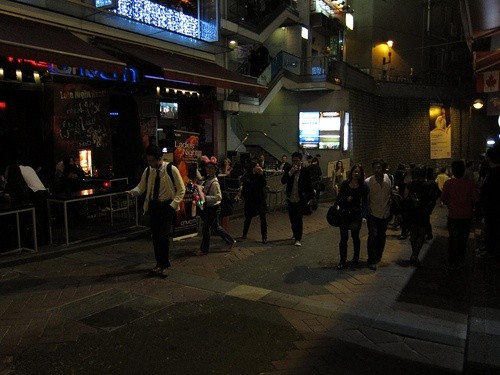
383, 38, 393, 65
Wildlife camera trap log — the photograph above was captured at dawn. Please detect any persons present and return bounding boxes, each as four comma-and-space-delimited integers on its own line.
249, 43, 274, 78
240, 159, 269, 244
257, 155, 268, 168
440, 158, 480, 248
306, 158, 322, 211
404, 163, 436, 265
332, 160, 346, 198
194, 155, 236, 256
19, 164, 49, 247
384, 155, 488, 240
363, 157, 395, 271
125, 145, 185, 276
276, 155, 291, 171
64, 154, 86, 189
476, 147, 500, 263
304, 155, 313, 168
314, 154, 321, 164
334, 164, 369, 271
281, 152, 313, 246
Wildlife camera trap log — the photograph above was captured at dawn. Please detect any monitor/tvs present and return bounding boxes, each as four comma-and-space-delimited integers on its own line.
160, 102, 178, 120
